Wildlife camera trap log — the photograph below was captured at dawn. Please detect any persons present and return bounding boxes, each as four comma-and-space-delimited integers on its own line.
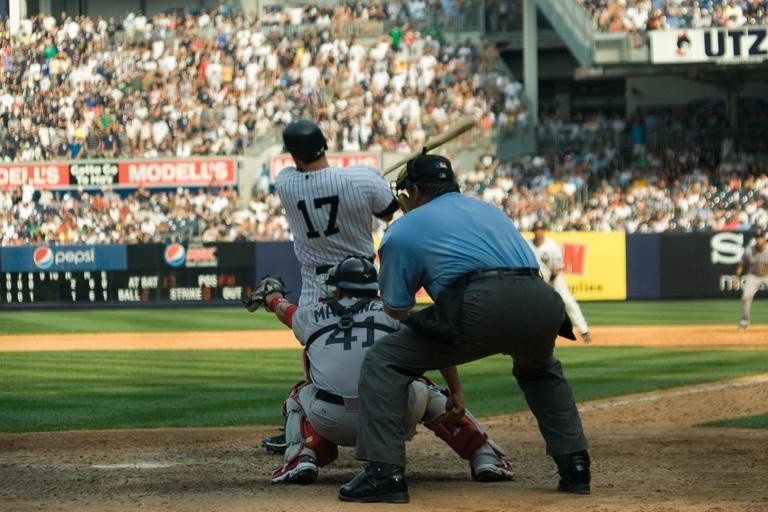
524, 221, 592, 345
730, 226, 768, 328
0, 1, 768, 236
337, 154, 592, 500
259, 119, 428, 457
244, 257, 518, 483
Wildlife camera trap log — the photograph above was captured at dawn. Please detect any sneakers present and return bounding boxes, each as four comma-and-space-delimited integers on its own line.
262, 434, 286, 452
470, 446, 515, 480
556, 449, 590, 493
339, 464, 410, 502
271, 455, 318, 485
582, 332, 590, 343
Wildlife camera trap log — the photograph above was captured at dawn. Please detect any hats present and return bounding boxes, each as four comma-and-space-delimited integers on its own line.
533, 220, 546, 231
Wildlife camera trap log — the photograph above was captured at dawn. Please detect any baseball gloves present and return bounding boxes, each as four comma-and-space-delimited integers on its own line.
246, 276, 284, 313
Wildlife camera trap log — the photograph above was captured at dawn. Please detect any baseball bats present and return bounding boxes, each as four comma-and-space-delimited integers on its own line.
380, 117, 474, 177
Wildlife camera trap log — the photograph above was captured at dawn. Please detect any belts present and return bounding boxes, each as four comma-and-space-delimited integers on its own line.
316, 258, 373, 275
467, 269, 539, 282
315, 390, 344, 406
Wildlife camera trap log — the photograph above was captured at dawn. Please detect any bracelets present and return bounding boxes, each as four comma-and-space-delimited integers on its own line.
549, 273, 557, 281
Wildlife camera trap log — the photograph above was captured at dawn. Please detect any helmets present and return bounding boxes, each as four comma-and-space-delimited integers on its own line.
283, 118, 328, 163
324, 256, 379, 298
751, 227, 764, 237
390, 155, 460, 213
